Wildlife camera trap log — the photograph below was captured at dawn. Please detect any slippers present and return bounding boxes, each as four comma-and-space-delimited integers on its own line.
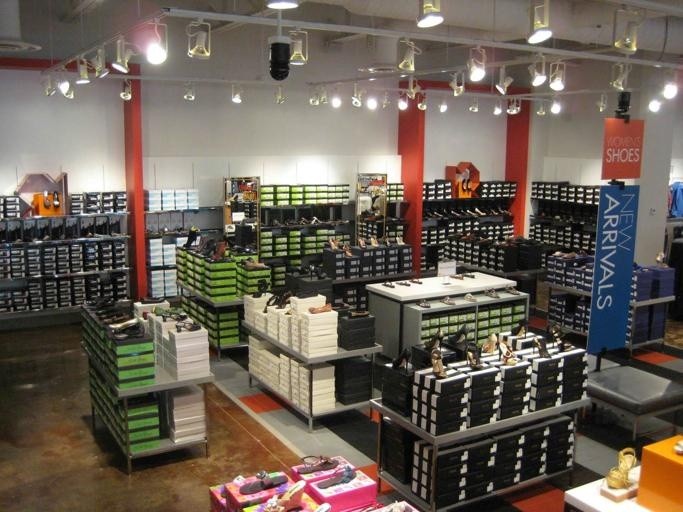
44, 190, 50, 208
221, 454, 413, 511
383, 270, 521, 308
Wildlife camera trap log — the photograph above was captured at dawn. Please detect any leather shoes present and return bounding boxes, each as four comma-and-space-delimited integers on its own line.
140, 297, 200, 332
423, 204, 513, 218
87, 296, 145, 339
448, 230, 588, 259
253, 263, 370, 318
53, 191, 60, 208
467, 179, 472, 191
463, 178, 467, 193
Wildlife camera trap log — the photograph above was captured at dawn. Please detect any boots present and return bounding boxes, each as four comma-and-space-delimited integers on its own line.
183, 230, 226, 261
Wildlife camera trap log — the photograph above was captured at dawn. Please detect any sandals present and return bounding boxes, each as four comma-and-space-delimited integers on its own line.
606, 447, 637, 490
232, 245, 270, 271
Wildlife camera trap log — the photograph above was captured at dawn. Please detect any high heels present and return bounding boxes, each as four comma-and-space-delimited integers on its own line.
391, 318, 572, 379
328, 234, 406, 257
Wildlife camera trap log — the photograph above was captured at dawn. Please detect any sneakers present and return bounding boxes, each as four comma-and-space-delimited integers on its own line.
0, 226, 93, 244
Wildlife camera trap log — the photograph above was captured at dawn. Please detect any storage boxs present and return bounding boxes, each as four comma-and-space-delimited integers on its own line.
208, 455, 377, 512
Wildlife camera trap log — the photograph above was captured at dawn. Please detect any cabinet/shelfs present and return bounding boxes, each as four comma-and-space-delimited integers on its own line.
369, 334, 590, 512
74, 296, 216, 477
0, 188, 199, 328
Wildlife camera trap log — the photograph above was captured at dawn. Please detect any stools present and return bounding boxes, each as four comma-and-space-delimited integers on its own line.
586, 366, 682, 444
